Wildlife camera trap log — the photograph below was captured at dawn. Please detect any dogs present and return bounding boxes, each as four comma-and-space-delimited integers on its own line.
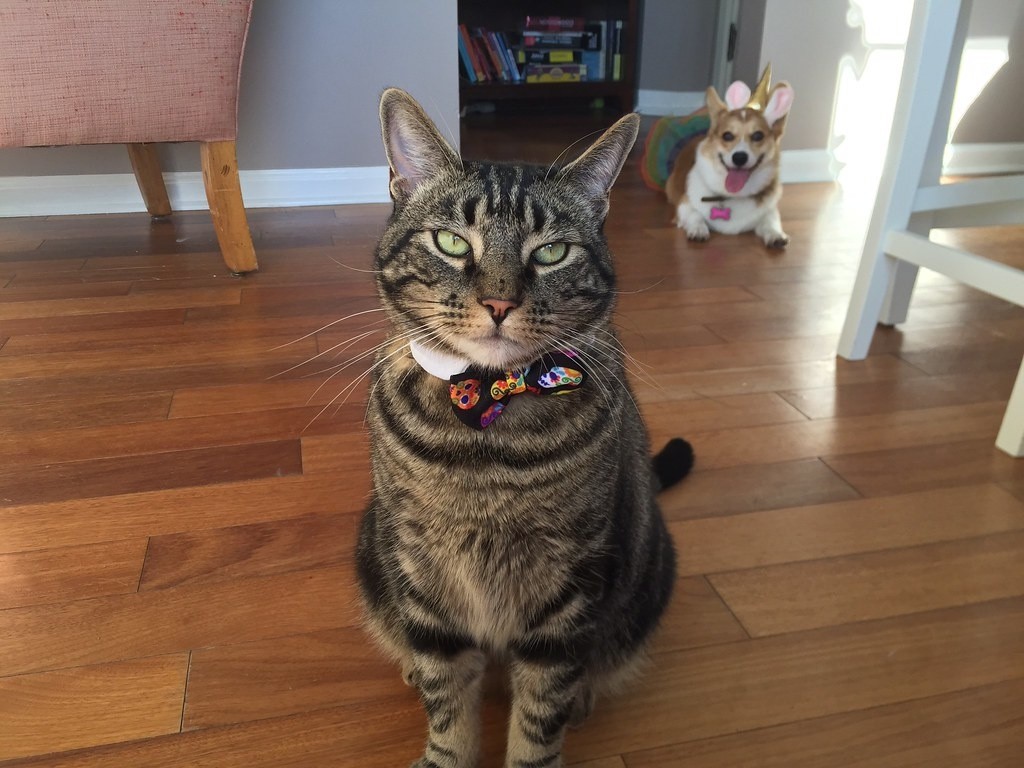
665, 81, 794, 249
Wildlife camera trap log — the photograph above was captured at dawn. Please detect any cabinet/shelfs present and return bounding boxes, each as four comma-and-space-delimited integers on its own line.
458, 0, 647, 123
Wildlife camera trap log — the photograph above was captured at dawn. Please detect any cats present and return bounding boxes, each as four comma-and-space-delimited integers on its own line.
348, 87, 697, 767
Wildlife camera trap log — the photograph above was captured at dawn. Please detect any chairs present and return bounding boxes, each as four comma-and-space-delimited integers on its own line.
2, 0, 266, 279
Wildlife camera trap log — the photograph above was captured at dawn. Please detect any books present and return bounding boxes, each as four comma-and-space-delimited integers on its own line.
456, 7, 624, 87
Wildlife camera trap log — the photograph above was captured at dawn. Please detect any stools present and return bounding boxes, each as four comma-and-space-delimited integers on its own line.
837, 6, 1024, 458
